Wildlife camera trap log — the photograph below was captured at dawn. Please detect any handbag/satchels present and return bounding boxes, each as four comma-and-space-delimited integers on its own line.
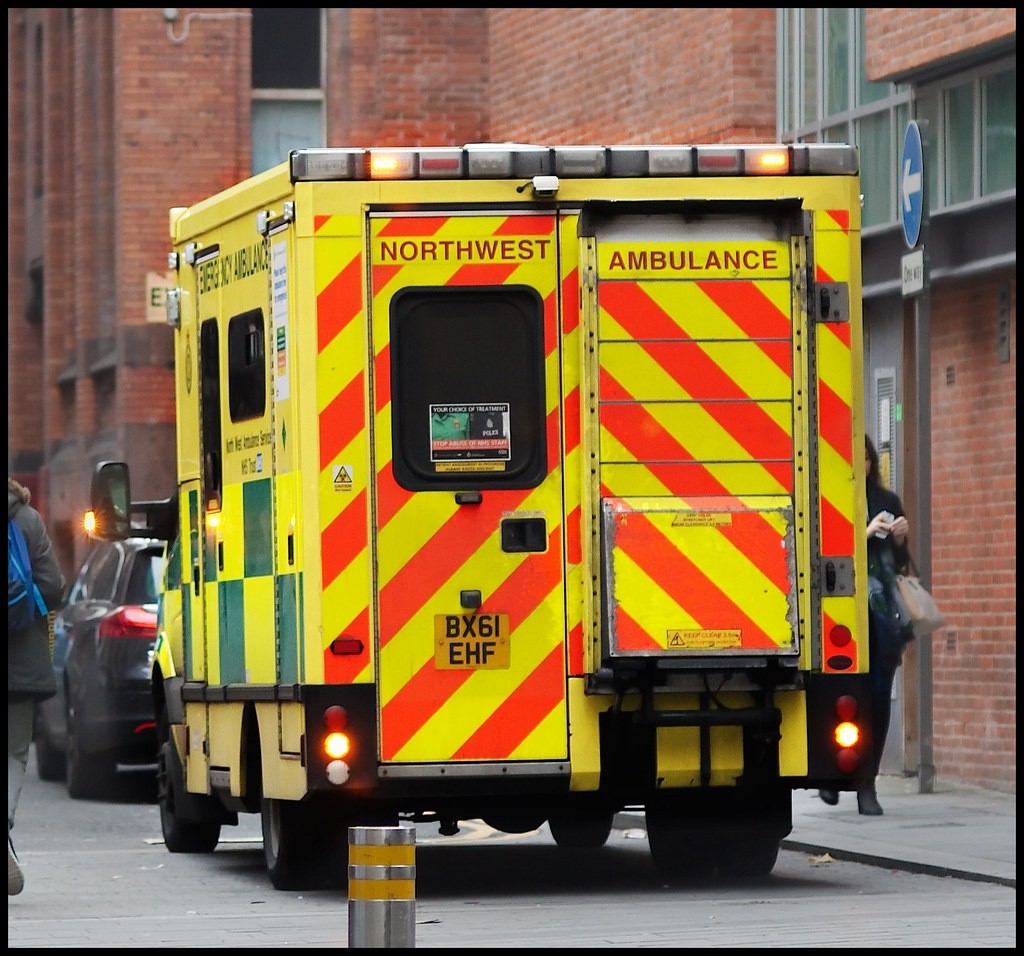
893, 531, 946, 636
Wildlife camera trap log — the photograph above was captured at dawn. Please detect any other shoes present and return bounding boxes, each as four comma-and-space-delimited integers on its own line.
8, 835, 25, 896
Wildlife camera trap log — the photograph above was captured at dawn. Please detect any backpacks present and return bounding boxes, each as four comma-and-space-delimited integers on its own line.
8, 521, 50, 633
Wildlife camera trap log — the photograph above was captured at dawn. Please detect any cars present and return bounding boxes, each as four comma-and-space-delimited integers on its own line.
35, 537, 164, 800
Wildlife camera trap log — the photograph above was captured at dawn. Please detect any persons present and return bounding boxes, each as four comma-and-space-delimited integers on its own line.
8, 477, 68, 896
820, 434, 916, 816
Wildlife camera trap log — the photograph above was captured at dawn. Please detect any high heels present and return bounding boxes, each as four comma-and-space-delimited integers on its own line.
857, 777, 883, 815
820, 791, 838, 805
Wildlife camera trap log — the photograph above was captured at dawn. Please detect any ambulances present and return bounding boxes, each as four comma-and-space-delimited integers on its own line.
92, 143, 868, 891
